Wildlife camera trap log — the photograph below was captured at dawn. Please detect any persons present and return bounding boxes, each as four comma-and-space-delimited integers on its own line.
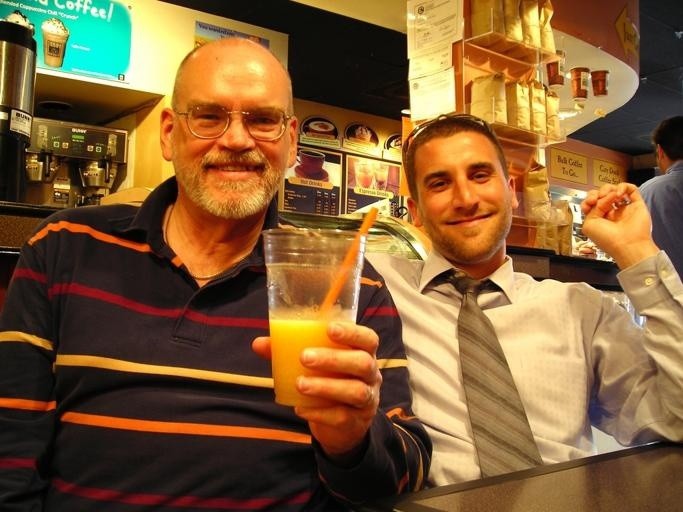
0, 36, 433, 512
363, 117, 683, 490
637, 115, 683, 284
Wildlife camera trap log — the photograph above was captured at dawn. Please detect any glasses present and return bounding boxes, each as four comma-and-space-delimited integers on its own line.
175, 103, 292, 143
401, 111, 490, 161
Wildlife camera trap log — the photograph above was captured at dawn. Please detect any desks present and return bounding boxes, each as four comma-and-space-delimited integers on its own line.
393, 440, 682, 512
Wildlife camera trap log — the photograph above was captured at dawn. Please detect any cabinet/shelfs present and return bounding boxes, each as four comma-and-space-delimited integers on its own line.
406, 0, 569, 254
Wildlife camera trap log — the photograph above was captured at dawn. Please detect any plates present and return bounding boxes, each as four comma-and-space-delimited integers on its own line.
295, 166, 328, 183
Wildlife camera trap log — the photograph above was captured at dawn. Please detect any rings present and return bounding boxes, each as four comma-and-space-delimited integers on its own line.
620, 196, 630, 206
354, 387, 375, 409
611, 202, 619, 209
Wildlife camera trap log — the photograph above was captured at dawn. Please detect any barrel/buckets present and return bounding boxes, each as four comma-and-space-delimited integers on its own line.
1, 21, 36, 125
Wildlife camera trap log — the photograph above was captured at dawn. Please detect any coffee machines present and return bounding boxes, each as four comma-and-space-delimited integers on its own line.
20, 118, 130, 212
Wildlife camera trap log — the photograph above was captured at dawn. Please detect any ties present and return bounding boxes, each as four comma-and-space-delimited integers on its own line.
432, 274, 545, 480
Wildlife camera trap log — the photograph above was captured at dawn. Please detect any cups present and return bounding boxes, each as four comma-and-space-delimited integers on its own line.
570, 67, 591, 101
546, 50, 565, 86
298, 149, 325, 174
303, 122, 335, 140
354, 162, 374, 190
40, 28, 67, 68
262, 229, 365, 409
590, 69, 609, 96
374, 166, 389, 194
